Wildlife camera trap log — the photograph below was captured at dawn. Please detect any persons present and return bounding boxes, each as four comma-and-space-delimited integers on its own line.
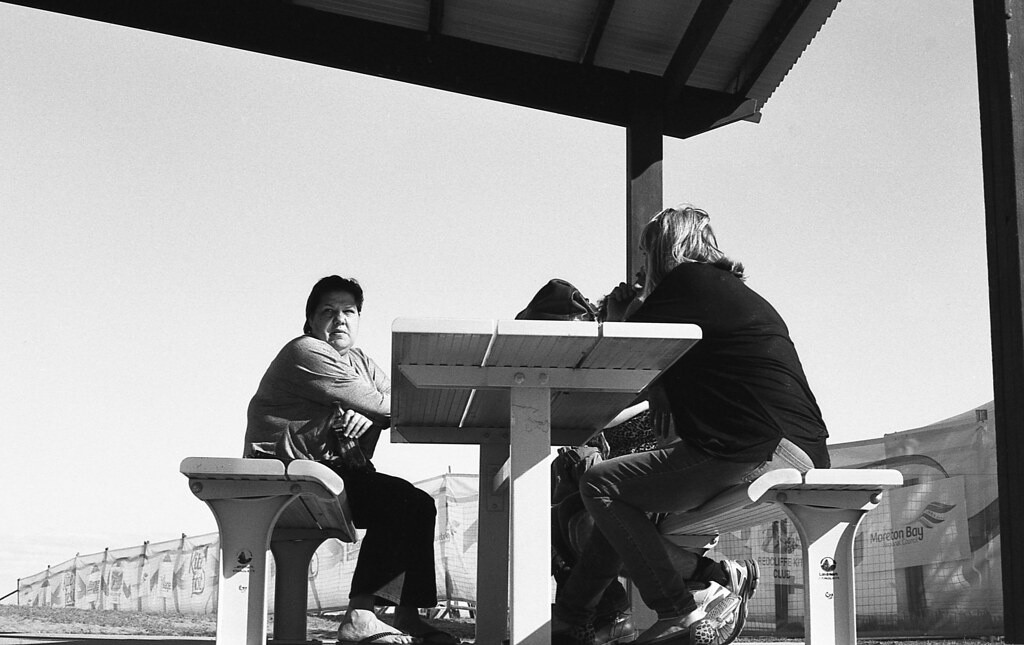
242, 275, 461, 645
550, 206, 828, 642
549, 284, 755, 641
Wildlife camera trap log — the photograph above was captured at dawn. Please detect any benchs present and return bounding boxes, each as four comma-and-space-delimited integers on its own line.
656, 468, 904, 644
181, 457, 358, 645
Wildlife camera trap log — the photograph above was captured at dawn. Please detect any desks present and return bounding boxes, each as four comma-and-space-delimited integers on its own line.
390, 316, 704, 644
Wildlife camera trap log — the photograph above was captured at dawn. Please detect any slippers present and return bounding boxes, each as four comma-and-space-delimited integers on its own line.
335, 628, 424, 645
411, 630, 461, 645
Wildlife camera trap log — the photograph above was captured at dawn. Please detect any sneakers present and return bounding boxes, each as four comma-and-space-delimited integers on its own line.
706, 557, 760, 645
552, 603, 595, 644
632, 609, 709, 645
594, 608, 637, 645
